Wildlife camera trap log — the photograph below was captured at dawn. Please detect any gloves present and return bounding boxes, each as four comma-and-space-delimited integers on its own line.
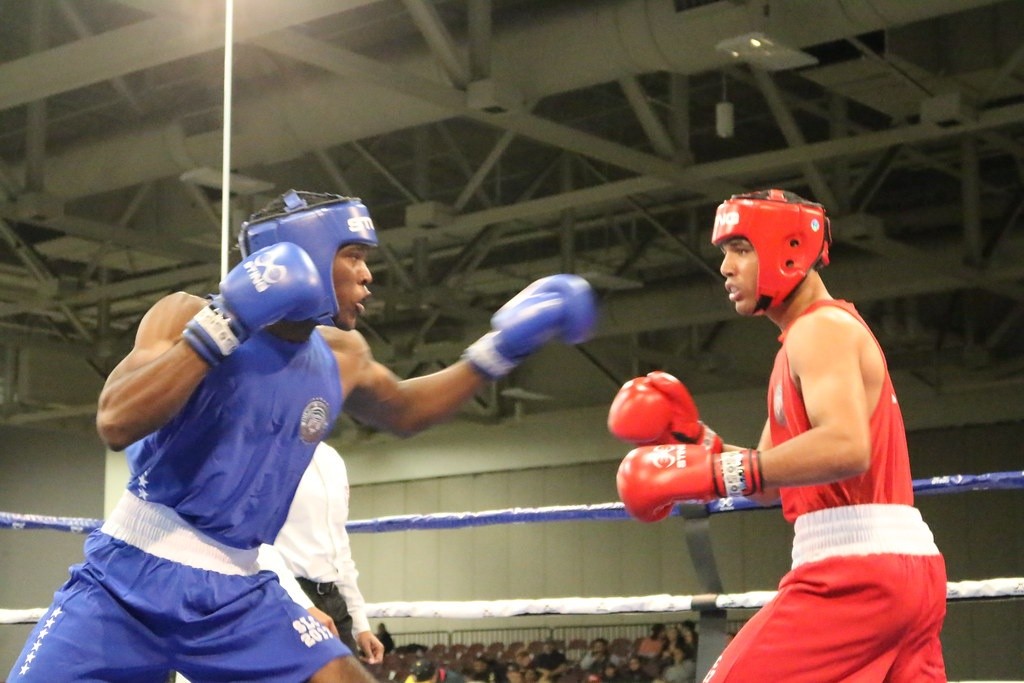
616, 444, 764, 522
607, 370, 723, 453
181, 242, 330, 368
465, 274, 598, 379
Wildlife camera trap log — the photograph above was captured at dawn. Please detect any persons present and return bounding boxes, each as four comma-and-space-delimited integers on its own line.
405, 622, 699, 683
0, 185, 596, 683
607, 187, 952, 683
375, 622, 395, 652
260, 438, 386, 669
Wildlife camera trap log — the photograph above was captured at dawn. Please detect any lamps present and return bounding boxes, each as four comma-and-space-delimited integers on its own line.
713, 30, 818, 72
179, 162, 277, 197
581, 271, 643, 290
714, 99, 733, 137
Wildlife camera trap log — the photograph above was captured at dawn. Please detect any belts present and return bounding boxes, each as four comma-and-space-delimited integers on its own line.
296, 578, 338, 595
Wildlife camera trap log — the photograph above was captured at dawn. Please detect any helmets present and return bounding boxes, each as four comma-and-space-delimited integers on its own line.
710, 189, 832, 315
238, 188, 379, 327
409, 658, 435, 681
